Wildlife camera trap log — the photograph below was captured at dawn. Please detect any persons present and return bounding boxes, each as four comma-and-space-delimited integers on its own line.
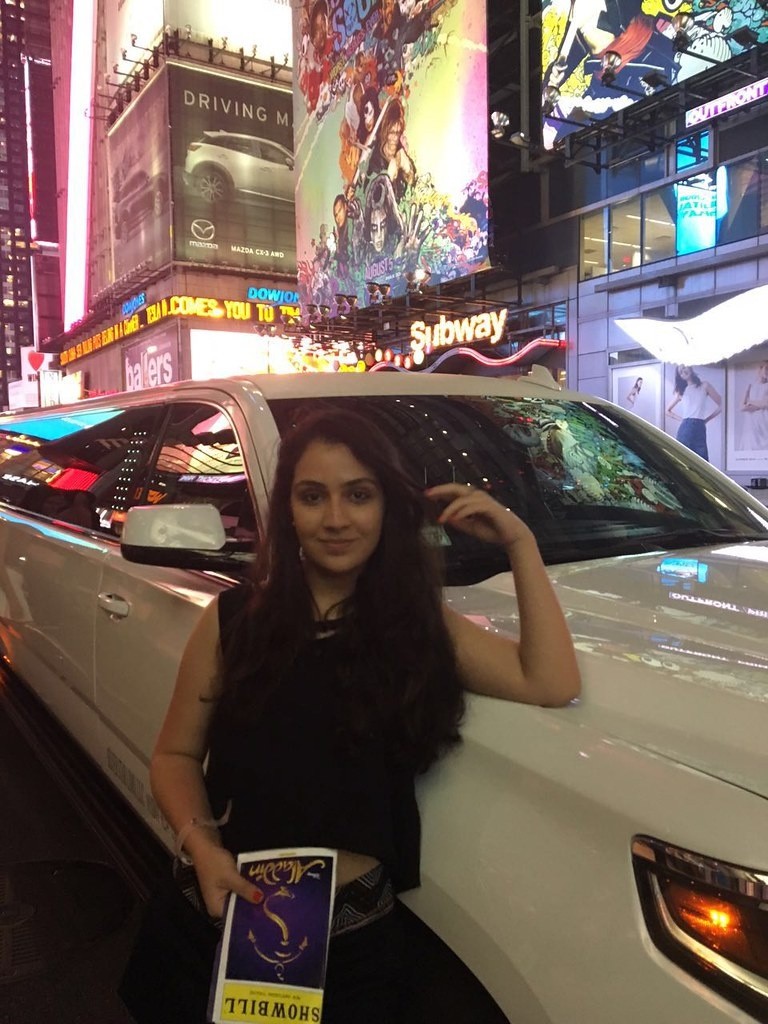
625, 376, 643, 412
149, 408, 582, 1024
742, 359, 768, 452
665, 363, 722, 463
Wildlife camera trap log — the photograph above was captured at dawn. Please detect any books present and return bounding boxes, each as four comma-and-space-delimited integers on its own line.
211, 847, 338, 1024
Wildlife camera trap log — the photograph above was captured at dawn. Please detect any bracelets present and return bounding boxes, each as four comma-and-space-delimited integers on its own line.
175, 816, 218, 866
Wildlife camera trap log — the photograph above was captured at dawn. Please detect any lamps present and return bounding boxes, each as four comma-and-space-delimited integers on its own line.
485, 110, 608, 170
538, 84, 649, 144
601, 51, 692, 112
672, 28, 758, 79
643, 67, 714, 103
80, 25, 289, 119
729, 25, 768, 50
259, 269, 521, 351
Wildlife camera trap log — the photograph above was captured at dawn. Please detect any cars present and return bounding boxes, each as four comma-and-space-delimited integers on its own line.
1, 372, 768, 1024
